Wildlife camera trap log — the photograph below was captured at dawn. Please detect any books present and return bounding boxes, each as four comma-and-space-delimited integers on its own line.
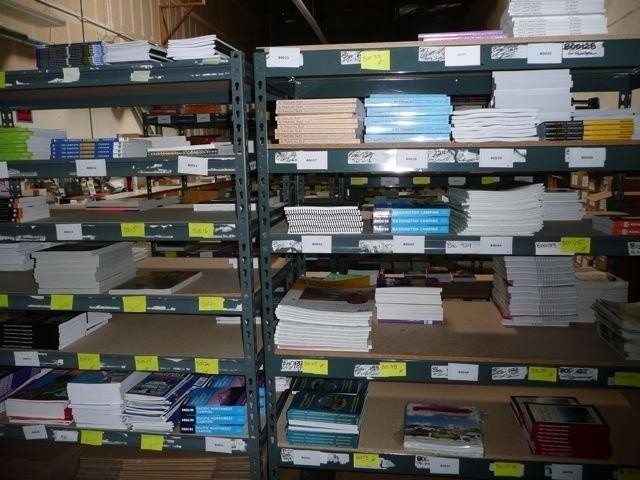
274, 271, 377, 353
282, 181, 639, 236
193, 198, 280, 212
402, 401, 485, 459
34, 33, 237, 69
213, 315, 262, 325
0, 367, 268, 437
108, 270, 203, 295
31, 242, 136, 294
490, 256, 628, 327
375, 275, 444, 325
85, 198, 158, 212
508, 395, 615, 461
0, 310, 112, 351
0, 195, 50, 224
591, 298, 640, 360
273, 67, 640, 143
0, 238, 67, 272
1, 125, 258, 159
283, 377, 370, 448
417, 0, 611, 41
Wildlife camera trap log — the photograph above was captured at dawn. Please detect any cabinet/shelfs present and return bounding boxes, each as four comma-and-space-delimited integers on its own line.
141, 112, 234, 259
253, 34, 640, 480
0, 52, 261, 480
